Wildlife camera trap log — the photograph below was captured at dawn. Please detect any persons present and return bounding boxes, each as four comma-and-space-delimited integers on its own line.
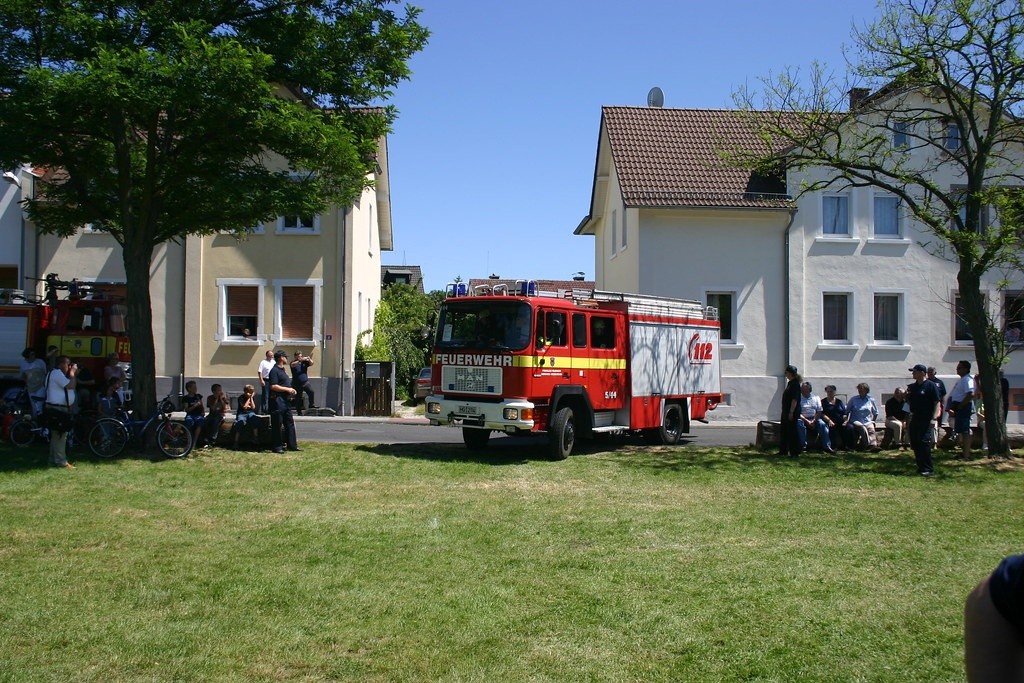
203, 383, 229, 449
242, 328, 250, 338
269, 350, 305, 453
181, 381, 205, 449
97, 352, 126, 445
235, 384, 256, 443
19, 347, 48, 415
45, 356, 80, 469
999, 368, 1009, 421
927, 367, 947, 427
976, 392, 988, 450
776, 365, 802, 457
820, 385, 855, 451
905, 364, 941, 477
290, 350, 319, 416
258, 350, 276, 414
885, 386, 913, 451
798, 382, 834, 452
847, 383, 878, 448
945, 361, 975, 459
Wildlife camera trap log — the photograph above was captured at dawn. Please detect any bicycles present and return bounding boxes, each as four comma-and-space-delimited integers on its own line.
86, 390, 194, 458
7, 390, 101, 449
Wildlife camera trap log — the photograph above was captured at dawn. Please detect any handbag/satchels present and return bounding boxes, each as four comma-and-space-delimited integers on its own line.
44, 408, 72, 432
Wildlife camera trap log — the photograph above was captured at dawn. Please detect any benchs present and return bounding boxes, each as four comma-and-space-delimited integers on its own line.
158, 412, 272, 431
874, 423, 977, 428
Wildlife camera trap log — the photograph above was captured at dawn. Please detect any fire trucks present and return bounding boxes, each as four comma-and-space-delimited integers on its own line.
420, 280, 724, 460
0, 273, 133, 399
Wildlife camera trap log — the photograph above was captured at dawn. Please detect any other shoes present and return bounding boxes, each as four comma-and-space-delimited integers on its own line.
841, 445, 853, 451
871, 446, 883, 453
899, 446, 904, 451
64, 462, 77, 469
285, 448, 304, 451
275, 448, 284, 454
921, 471, 929, 476
824, 447, 836, 455
298, 411, 303, 416
907, 446, 910, 450
308, 405, 319, 408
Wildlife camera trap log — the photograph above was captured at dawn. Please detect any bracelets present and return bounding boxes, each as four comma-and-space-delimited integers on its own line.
933, 417, 937, 420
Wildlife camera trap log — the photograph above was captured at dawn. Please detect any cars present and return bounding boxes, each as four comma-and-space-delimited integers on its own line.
412, 367, 431, 402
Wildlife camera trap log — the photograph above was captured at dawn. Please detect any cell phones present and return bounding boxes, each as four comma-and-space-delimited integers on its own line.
303, 357, 310, 361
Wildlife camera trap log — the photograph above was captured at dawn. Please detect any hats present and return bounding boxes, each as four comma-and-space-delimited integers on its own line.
785, 365, 797, 374
274, 350, 289, 358
909, 364, 926, 374
